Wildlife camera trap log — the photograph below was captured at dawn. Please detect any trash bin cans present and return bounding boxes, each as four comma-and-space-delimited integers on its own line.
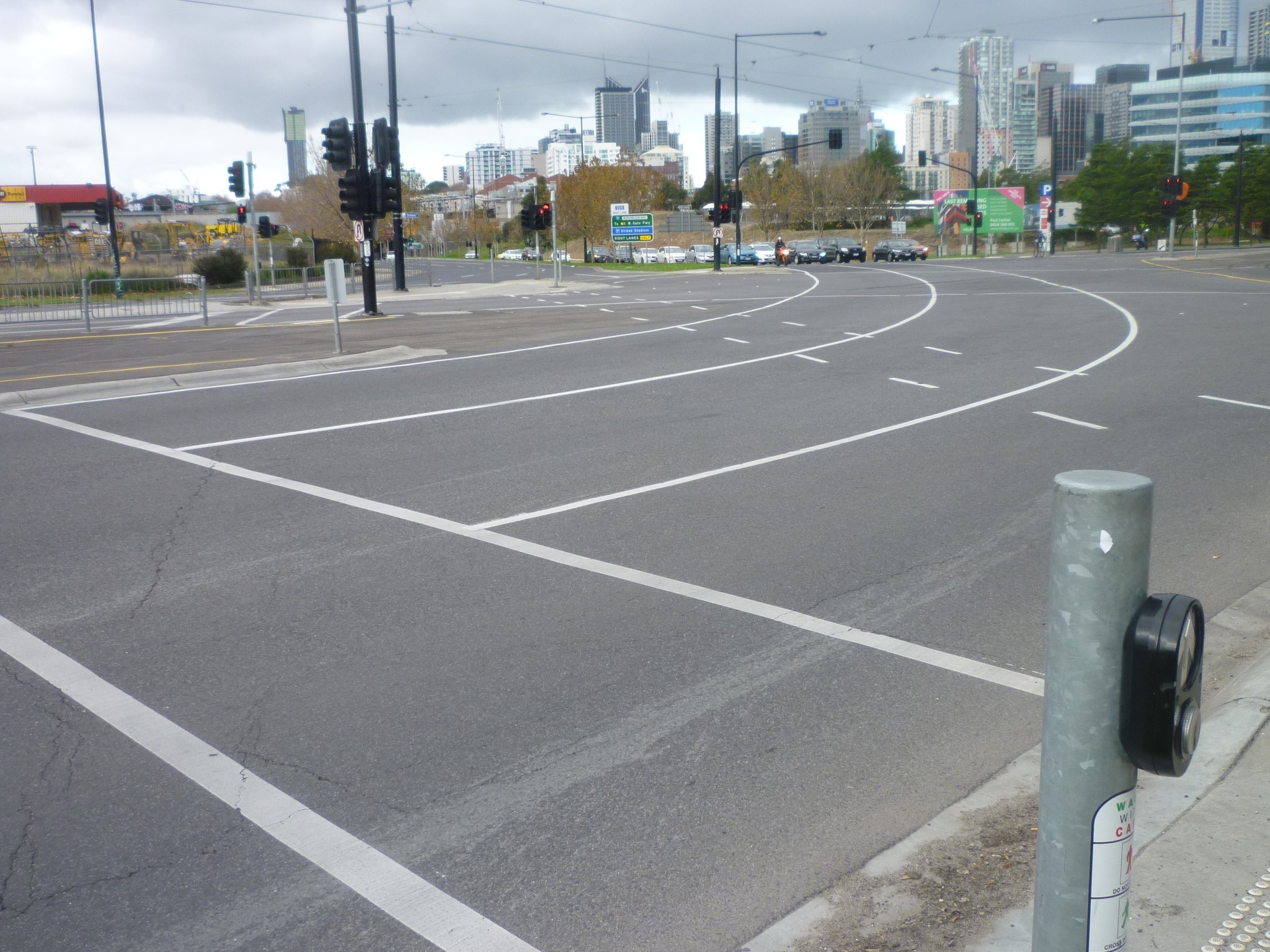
1107, 236, 1123, 252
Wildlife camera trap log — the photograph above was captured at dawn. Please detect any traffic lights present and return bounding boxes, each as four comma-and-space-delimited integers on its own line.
336, 169, 362, 220
227, 160, 244, 198
319, 115, 353, 171
543, 202, 552, 226
520, 208, 529, 228
259, 215, 272, 239
974, 212, 983, 227
372, 167, 400, 218
966, 218, 971, 226
236, 205, 247, 224
966, 200, 974, 215
1047, 207, 1053, 222
1162, 193, 1175, 206
1162, 175, 1182, 195
373, 117, 399, 168
529, 205, 545, 230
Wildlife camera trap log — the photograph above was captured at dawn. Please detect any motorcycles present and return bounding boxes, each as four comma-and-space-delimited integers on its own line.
775, 247, 789, 267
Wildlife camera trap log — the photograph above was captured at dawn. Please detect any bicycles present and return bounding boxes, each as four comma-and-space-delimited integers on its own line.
1033, 242, 1045, 259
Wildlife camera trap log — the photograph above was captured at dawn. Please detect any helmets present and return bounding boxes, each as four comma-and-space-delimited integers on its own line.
777, 237, 781, 240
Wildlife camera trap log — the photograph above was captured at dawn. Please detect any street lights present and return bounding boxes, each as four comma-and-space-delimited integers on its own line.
444, 153, 508, 259
733, 29, 828, 274
1090, 11, 1187, 258
931, 65, 980, 254
541, 111, 620, 264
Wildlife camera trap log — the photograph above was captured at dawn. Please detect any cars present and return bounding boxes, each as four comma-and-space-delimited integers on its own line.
748, 243, 775, 263
633, 248, 658, 264
521, 247, 543, 262
551, 249, 571, 262
871, 237, 928, 262
719, 242, 759, 265
503, 250, 521, 260
606, 250, 630, 263
587, 246, 609, 263
786, 235, 867, 263
655, 246, 687, 264
685, 245, 714, 263
465, 250, 479, 259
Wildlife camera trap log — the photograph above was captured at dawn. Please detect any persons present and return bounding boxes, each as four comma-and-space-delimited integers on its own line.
774, 236, 785, 259
1134, 221, 1150, 238
1034, 230, 1046, 256
